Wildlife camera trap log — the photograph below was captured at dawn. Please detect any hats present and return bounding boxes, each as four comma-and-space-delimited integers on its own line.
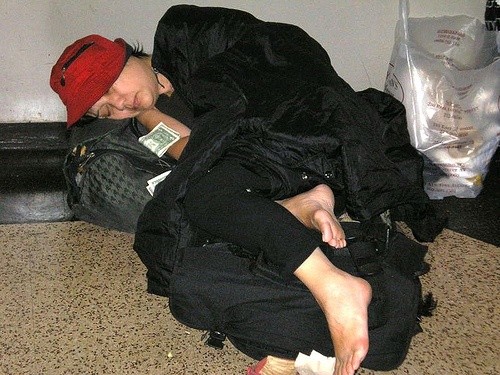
50, 34, 127, 129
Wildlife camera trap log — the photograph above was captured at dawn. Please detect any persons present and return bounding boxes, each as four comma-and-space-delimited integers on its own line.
50, 4, 373, 375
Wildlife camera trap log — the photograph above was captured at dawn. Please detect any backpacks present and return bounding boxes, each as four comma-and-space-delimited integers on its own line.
170, 220, 422, 370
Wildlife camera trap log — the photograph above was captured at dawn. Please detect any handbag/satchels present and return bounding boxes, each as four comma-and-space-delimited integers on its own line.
385, 0, 500, 200
63, 117, 173, 234
